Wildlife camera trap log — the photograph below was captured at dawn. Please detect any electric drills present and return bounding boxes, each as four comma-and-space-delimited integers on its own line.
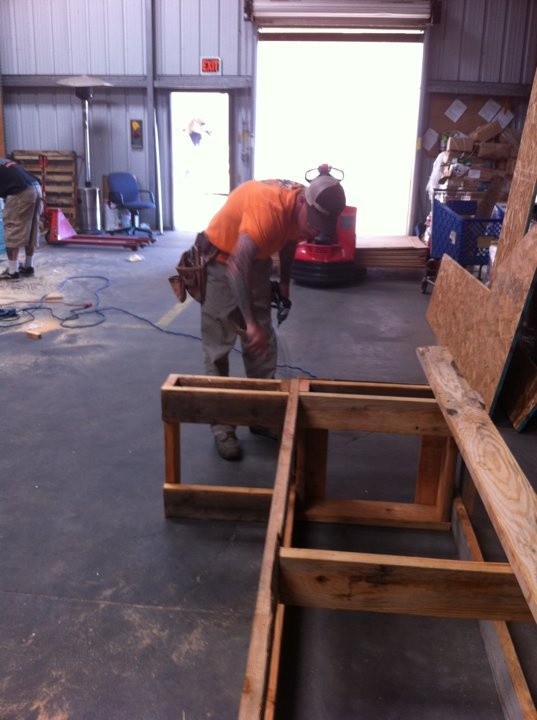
267, 281, 292, 328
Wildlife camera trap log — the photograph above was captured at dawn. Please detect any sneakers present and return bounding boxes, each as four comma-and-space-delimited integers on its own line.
248, 424, 281, 440
0, 268, 19, 281
18, 263, 34, 275
215, 431, 240, 460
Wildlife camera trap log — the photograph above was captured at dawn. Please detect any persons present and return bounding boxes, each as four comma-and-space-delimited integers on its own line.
0, 158, 45, 282
182, 174, 347, 461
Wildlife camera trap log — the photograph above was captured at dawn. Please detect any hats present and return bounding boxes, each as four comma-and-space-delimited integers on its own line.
303, 174, 346, 245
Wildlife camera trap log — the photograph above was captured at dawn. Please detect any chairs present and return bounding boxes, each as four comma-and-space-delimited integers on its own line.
106, 173, 158, 249
